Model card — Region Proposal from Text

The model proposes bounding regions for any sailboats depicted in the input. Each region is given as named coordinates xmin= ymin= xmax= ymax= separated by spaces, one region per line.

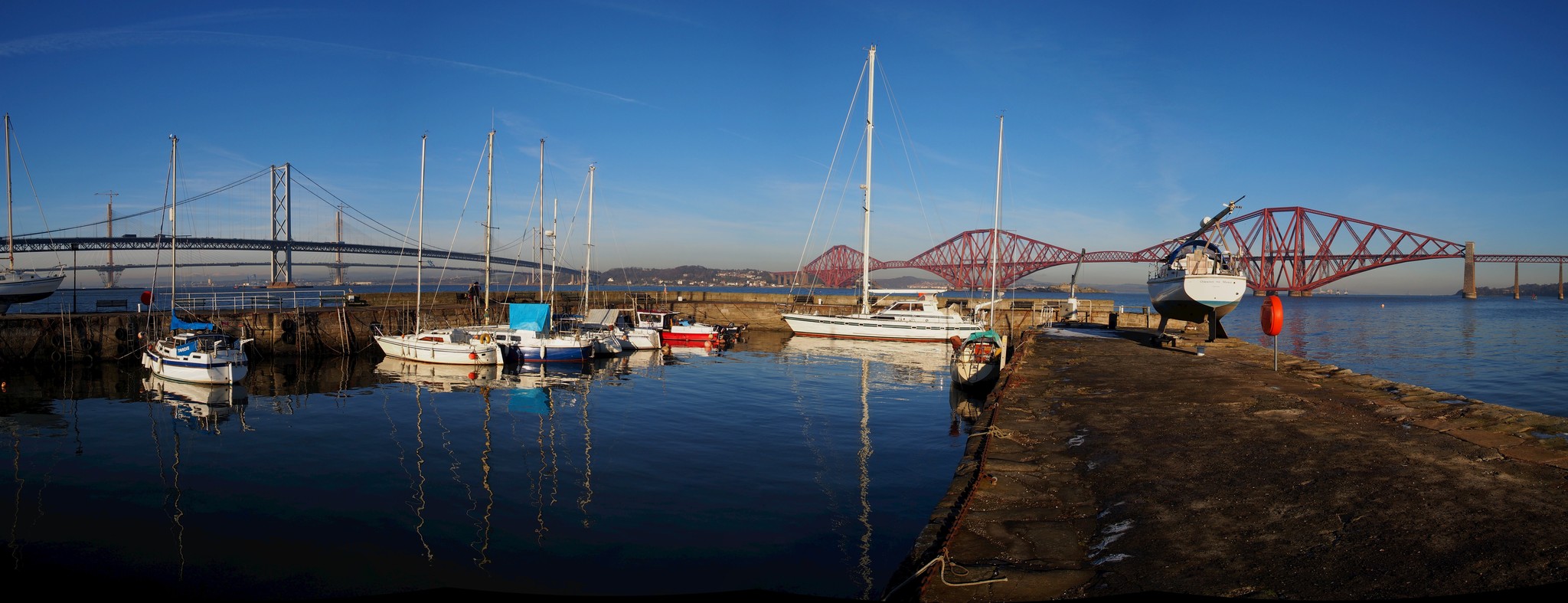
xmin=141 ymin=134 xmax=256 ymax=384
xmin=0 ymin=113 xmax=67 ymax=306
xmin=780 ymin=41 xmax=990 ymax=344
xmin=372 ymin=128 xmax=741 ymax=367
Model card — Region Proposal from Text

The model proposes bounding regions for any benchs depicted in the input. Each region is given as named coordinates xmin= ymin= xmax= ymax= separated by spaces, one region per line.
xmin=945 ymin=299 xmax=968 ymax=309
xmin=793 ymin=295 xmax=814 ymax=304
xmin=456 ymin=293 xmax=481 ymax=304
xmin=1010 ymin=301 xmax=1034 ymax=309
xmin=319 ymin=295 xmax=348 ymax=308
xmin=250 ymin=297 xmax=283 ymax=310
xmin=96 ymin=299 xmax=128 ymax=312
xmin=561 ymin=293 xmax=578 ymax=302
xmin=171 ymin=298 xmax=206 ymax=310
xmin=857 ymin=296 xmax=878 ymax=305
xmin=511 ymin=292 xmax=535 ymax=303
xmin=630 ymin=292 xmax=647 ymax=299
xmin=1044 ymin=301 xmax=1067 ymax=308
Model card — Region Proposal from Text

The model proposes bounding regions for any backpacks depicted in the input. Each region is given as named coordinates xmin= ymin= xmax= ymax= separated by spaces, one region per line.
xmin=464 ymin=293 xmax=468 ymax=299
xmin=460 ymin=294 xmax=464 ymax=299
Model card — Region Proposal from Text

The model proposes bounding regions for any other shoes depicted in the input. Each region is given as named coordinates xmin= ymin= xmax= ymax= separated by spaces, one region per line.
xmin=479 ymin=303 xmax=481 ymax=305
xmin=474 ymin=304 xmax=477 ymax=306
xmin=469 ymin=304 xmax=473 ymax=305
xmin=477 ymin=304 xmax=479 ymax=306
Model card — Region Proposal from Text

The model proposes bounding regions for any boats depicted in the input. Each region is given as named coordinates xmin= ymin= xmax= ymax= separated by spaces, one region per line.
xmin=1147 ymin=227 xmax=1248 ymax=322
xmin=949 ymin=330 xmax=1007 ymax=387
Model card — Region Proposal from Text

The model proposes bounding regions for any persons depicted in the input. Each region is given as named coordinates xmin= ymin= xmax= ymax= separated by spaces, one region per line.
xmin=468 ymin=282 xmax=481 ymax=306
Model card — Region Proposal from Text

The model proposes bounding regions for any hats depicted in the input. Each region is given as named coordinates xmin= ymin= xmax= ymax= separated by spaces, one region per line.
xmin=476 ymin=281 xmax=478 ymax=284
xmin=470 ymin=284 xmax=473 ymax=287
xmin=474 ymin=283 xmax=476 ymax=285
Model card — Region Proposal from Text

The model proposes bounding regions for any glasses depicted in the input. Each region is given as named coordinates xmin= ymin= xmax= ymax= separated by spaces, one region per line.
xmin=469 ymin=286 xmax=471 ymax=286
